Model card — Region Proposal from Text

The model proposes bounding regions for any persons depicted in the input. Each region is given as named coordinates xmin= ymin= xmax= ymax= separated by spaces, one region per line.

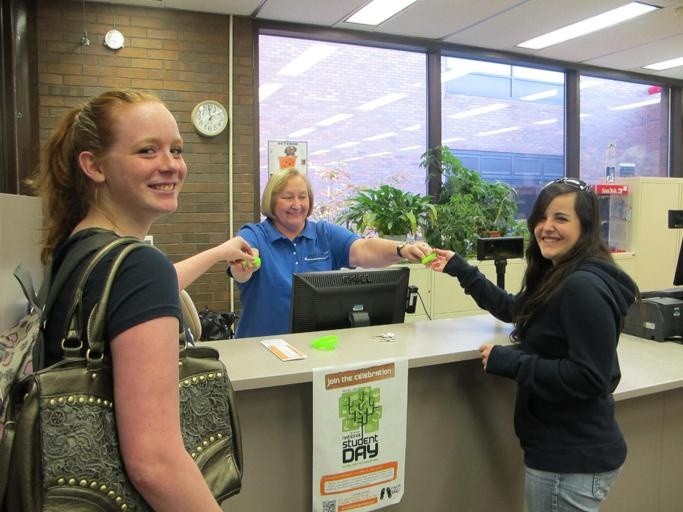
xmin=283 ymin=146 xmax=305 ymax=171
xmin=225 ymin=166 xmax=435 ymax=340
xmin=430 ymin=177 xmax=643 ymax=510
xmin=0 ymin=88 xmax=256 ymax=512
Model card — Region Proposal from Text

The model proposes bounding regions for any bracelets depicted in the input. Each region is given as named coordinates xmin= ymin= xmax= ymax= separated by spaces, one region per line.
xmin=242 ymin=254 xmax=262 ymax=270
xmin=421 ymin=251 xmax=437 ymax=265
xmin=311 ymin=335 xmax=339 ymax=351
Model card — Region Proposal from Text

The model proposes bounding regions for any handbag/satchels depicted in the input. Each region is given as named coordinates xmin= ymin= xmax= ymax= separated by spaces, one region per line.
xmin=1 ymin=234 xmax=244 ymax=512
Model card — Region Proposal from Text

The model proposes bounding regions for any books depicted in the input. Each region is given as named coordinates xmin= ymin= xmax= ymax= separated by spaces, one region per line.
xmin=260 ymin=338 xmax=307 ymax=361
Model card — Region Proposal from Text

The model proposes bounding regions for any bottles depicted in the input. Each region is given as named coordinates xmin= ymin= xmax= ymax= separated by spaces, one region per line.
xmin=605 ymin=144 xmax=615 ymax=185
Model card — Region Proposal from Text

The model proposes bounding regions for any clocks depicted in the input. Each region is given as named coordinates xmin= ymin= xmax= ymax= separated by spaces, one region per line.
xmin=192 ymin=100 xmax=228 ymax=140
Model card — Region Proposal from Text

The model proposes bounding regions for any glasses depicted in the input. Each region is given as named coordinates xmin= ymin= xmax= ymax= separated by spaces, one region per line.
xmin=538 ymin=177 xmax=593 ymax=196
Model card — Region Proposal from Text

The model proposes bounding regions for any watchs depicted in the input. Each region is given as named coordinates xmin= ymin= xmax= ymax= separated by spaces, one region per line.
xmin=397 ymin=241 xmax=409 ymax=258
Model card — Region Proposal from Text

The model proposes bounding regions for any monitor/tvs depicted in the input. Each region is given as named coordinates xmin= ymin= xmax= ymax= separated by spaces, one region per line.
xmin=291 ymin=267 xmax=409 ymax=332
